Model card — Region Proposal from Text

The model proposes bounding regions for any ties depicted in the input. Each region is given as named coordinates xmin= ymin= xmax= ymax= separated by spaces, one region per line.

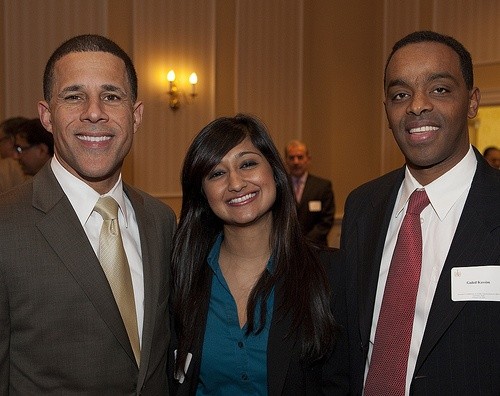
xmin=363 ymin=191 xmax=430 ymax=396
xmin=294 ymin=179 xmax=303 ymax=202
xmin=94 ymin=196 xmax=141 ymax=369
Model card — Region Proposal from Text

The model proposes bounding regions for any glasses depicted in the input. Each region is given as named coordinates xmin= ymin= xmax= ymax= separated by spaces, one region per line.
xmin=14 ymin=144 xmax=35 ymax=156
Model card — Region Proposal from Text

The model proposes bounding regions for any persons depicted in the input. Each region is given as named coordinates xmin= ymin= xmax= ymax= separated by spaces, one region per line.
xmin=0 ymin=116 xmax=34 ymax=193
xmin=162 ymin=111 xmax=343 ymax=396
xmin=11 ymin=118 xmax=55 ymax=176
xmin=282 ymin=140 xmax=335 ymax=247
xmin=330 ymin=27 xmax=500 ymax=396
xmin=0 ymin=33 xmax=181 ymax=396
xmin=482 ymin=146 xmax=500 ymax=170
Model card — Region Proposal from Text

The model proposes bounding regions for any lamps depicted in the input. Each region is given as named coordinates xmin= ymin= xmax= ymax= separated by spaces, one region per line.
xmin=165 ymin=69 xmax=199 ymax=106
